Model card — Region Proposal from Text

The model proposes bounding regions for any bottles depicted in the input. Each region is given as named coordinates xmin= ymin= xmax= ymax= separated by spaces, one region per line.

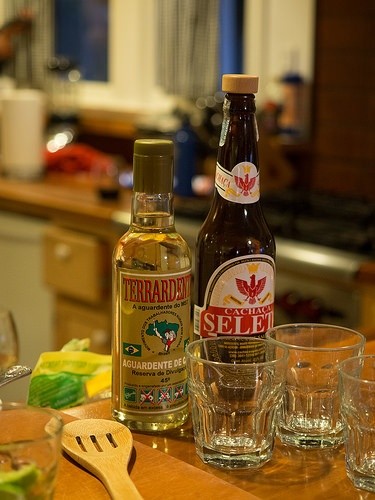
xmin=110 ymin=138 xmax=192 ymax=435
xmin=195 ymin=73 xmax=277 ymax=418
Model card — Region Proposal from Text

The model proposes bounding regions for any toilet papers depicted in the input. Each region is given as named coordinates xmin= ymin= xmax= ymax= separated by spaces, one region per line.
xmin=0 ymin=89 xmax=46 ymax=176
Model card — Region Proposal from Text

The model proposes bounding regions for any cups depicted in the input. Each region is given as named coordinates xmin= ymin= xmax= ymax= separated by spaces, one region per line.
xmin=0 ymin=310 xmax=18 ymax=373
xmin=0 ymin=403 xmax=65 ymax=500
xmin=265 ymin=323 xmax=366 ymax=450
xmin=336 ymin=354 xmax=375 ymax=494
xmin=185 ymin=337 xmax=289 ymax=470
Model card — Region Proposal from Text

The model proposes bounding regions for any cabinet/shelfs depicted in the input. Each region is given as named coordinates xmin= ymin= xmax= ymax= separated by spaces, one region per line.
xmin=39 ymin=222 xmax=114 ymax=352
